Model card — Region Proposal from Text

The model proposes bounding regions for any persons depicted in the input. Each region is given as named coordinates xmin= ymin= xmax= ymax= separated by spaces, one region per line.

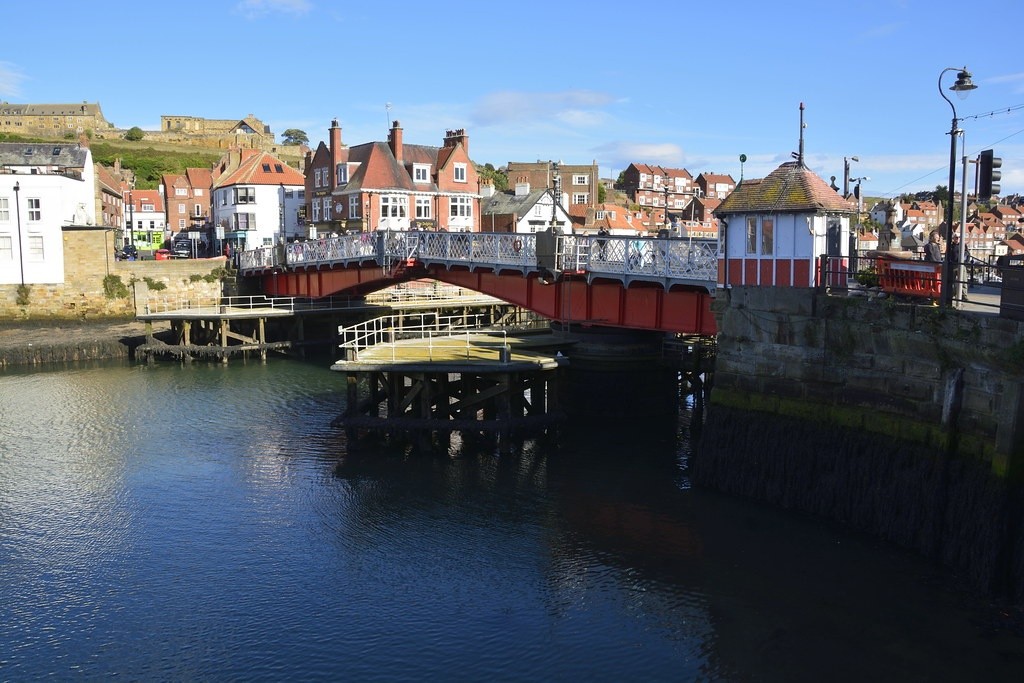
xmin=254 ymin=226 xmax=472 ymax=267
xmin=945 ymin=233 xmax=968 ymax=301
xmin=924 ymin=231 xmax=942 ymax=262
xmin=596 ymin=226 xmax=610 ymax=262
xmin=632 ymin=231 xmax=650 ymax=270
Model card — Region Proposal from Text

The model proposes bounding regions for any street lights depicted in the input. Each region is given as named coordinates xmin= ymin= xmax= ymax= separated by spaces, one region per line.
xmin=844 ymin=156 xmax=859 ymax=198
xmin=476 ymin=177 xmax=481 ymax=232
xmin=366 ymin=191 xmax=373 ymax=233
xmin=938 ymin=65 xmax=978 ymax=301
xmin=434 ymin=195 xmax=439 ymax=232
xmin=128 ymin=182 xmax=135 ymax=245
xmin=13 ymin=181 xmax=24 ymax=286
xmin=552 ymin=171 xmax=561 ymax=227
xmin=848 ymin=176 xmax=870 ymax=279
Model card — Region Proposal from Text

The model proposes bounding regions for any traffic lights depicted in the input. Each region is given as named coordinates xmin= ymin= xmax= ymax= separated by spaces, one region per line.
xmin=979 ymin=149 xmax=1002 ymax=200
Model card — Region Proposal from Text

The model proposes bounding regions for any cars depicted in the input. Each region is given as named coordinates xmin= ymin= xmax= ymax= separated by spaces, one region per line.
xmin=120 ymin=245 xmax=138 ymax=259
xmin=154 ymin=249 xmax=171 ymax=260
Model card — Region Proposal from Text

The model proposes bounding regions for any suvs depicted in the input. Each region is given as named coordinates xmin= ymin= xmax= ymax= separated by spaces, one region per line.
xmin=173 ymin=240 xmax=206 ymax=259
